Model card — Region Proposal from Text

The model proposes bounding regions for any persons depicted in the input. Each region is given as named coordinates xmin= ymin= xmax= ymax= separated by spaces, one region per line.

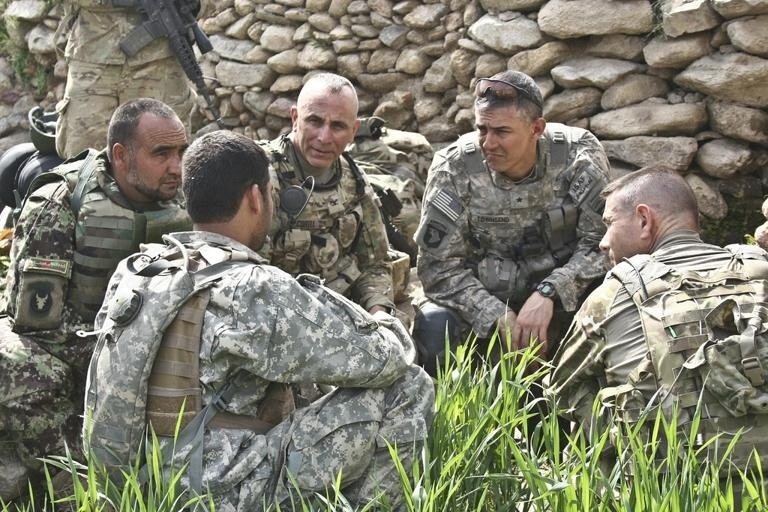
xmin=541 ymin=164 xmax=768 ymax=502
xmin=43 ymin=0 xmax=195 ymax=159
xmin=412 ymin=70 xmax=612 ymax=382
xmin=250 ymin=73 xmax=411 ymax=331
xmin=0 ymin=314 xmax=87 ymax=509
xmin=6 ymin=98 xmax=194 ymax=381
xmin=80 ymin=130 xmax=436 ymax=512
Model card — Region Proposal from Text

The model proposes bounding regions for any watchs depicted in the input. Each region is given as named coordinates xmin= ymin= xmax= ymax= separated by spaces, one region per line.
xmin=533 ymin=283 xmax=560 ymax=303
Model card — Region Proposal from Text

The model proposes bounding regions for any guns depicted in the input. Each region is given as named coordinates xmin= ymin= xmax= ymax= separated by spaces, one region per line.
xmin=114 ymin=0 xmax=224 ymax=129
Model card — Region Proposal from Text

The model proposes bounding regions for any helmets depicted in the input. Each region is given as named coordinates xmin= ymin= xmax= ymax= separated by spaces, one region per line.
xmin=26 ymin=105 xmax=58 ymax=155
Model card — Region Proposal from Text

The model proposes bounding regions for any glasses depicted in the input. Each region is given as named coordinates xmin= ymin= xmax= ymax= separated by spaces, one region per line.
xmin=473 ymin=77 xmax=542 ymax=110
